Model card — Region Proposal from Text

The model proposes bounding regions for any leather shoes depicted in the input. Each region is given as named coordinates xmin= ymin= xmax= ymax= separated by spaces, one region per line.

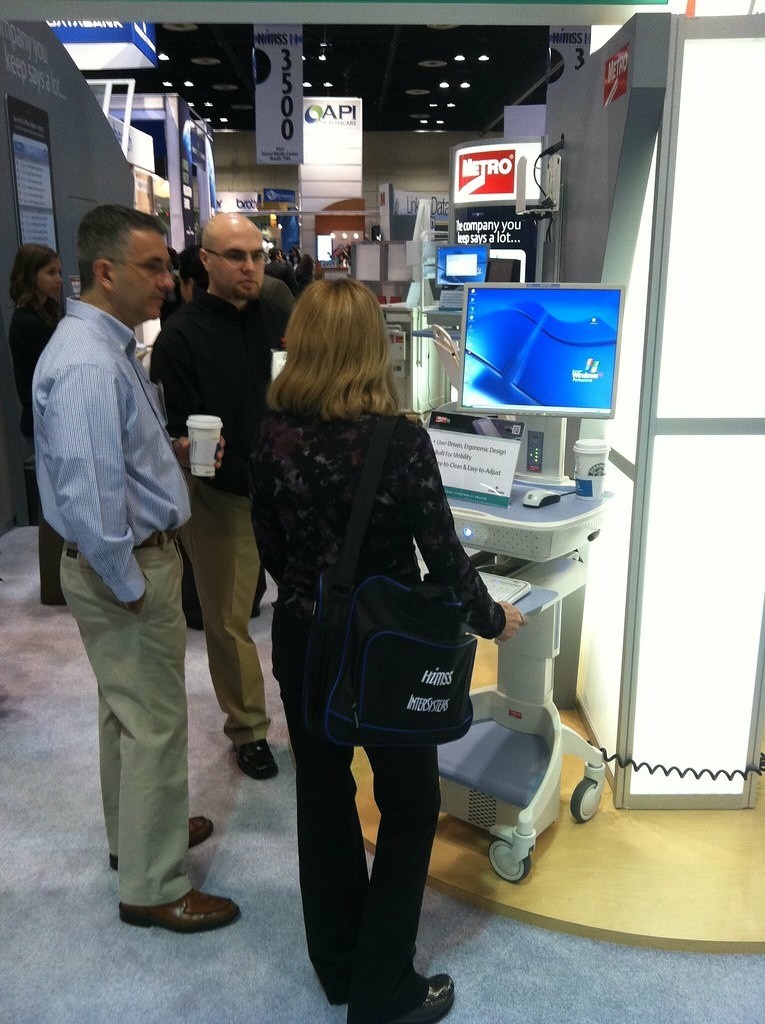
xmin=119 ymin=889 xmax=240 ymax=934
xmin=233 ymin=739 xmax=279 ymax=779
xmin=390 ymin=973 xmax=455 ymax=1024
xmin=109 ymin=815 xmax=213 ymax=870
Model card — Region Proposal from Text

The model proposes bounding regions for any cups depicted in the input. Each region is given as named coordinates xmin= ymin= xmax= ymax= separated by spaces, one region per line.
xmin=186 ymin=414 xmax=223 ymax=477
xmin=272 ymin=351 xmax=287 ymax=382
xmin=573 ymin=438 xmax=611 ymax=502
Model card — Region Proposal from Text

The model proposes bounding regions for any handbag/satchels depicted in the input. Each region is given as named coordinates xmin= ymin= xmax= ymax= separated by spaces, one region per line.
xmin=304 ymin=572 xmax=478 ymax=748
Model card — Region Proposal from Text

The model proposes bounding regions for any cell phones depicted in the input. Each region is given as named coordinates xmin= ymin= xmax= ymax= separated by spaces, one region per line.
xmin=3 ymin=94 xmax=61 ymax=256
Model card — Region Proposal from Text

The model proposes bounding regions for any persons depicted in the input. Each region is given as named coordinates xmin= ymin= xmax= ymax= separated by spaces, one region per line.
xmin=331 ymin=245 xmax=351 ymax=267
xmin=134 ymin=229 xmax=316 ymax=631
xmin=32 ymin=203 xmax=242 ymax=933
xmin=149 ymin=211 xmax=281 ymax=782
xmin=186 ymin=225 xmax=195 ymax=235
xmin=245 ymin=277 xmax=531 ymax=1024
xmin=9 ymin=241 xmax=64 ymax=609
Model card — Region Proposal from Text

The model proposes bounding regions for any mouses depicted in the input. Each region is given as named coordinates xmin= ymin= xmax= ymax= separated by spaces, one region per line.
xmin=522 ymin=489 xmax=561 ymax=508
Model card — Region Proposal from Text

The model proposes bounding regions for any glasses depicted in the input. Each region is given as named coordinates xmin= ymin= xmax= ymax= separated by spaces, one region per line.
xmin=203 ymin=248 xmax=271 ymax=267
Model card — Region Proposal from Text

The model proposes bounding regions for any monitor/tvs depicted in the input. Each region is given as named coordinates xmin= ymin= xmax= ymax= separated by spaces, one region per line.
xmin=456 ymin=282 xmax=627 ymax=419
xmin=434 ymin=243 xmax=490 ymax=288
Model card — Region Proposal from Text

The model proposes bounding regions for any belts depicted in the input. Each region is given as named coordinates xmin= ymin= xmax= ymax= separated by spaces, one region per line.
xmin=134 ymin=528 xmax=178 ymax=550
